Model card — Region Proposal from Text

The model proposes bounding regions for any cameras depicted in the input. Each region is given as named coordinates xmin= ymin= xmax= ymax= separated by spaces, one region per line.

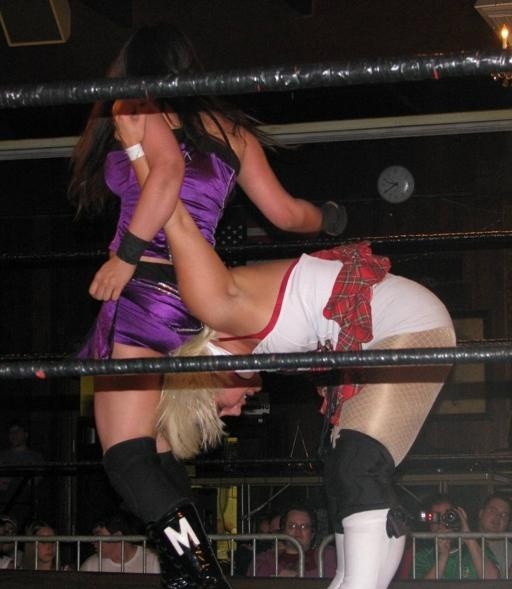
xmin=415 ymin=508 xmax=461 ymax=527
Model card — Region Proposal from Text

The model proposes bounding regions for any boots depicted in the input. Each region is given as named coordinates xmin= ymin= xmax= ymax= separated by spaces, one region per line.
xmin=145 ymin=497 xmax=232 ymax=587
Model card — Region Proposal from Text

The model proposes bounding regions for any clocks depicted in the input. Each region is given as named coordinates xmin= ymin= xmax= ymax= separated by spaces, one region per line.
xmin=377 ymin=165 xmax=415 ymax=204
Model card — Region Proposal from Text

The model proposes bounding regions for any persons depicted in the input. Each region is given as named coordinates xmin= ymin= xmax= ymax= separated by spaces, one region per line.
xmin=236 ymin=503 xmax=337 ymax=579
xmin=65 ymin=19 xmax=350 ymax=589
xmin=395 ymin=490 xmax=512 ymax=579
xmin=111 ymin=97 xmax=458 ymax=589
xmin=0 ymin=516 xmax=162 ymax=573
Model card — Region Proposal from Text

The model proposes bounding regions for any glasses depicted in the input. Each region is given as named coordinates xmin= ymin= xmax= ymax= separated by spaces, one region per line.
xmin=284 ymin=522 xmax=314 ymax=532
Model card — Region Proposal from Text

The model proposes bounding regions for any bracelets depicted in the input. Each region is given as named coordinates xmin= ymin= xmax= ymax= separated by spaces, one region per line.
xmin=123 ymin=141 xmax=145 ymax=161
xmin=117 ymin=228 xmax=150 ymax=266
